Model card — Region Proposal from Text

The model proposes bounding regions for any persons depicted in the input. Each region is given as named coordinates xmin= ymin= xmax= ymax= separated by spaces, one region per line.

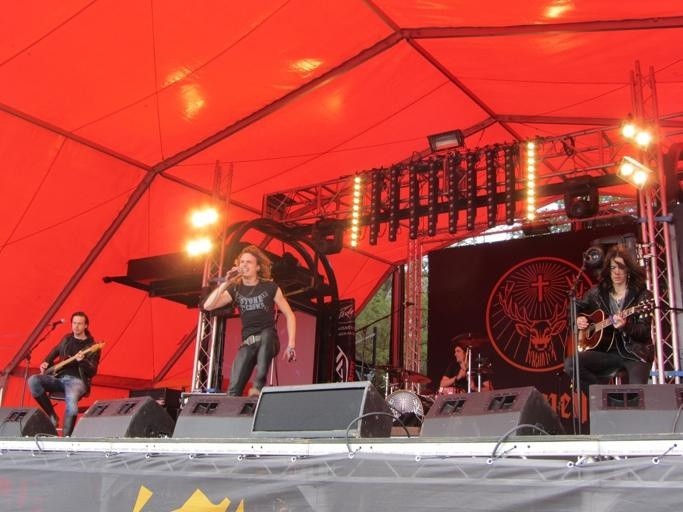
xmin=203 ymin=246 xmax=296 ymax=397
xmin=564 ymin=245 xmax=655 ymax=400
xmin=440 ymin=343 xmax=494 ymax=392
xmin=28 ymin=312 xmax=101 ymax=437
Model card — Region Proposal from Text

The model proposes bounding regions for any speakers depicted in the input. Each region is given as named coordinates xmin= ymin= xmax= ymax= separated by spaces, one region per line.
xmin=68 ymin=397 xmax=175 ymax=438
xmin=420 ymin=385 xmax=563 ymax=437
xmin=588 ymin=383 xmax=683 ymax=435
xmin=251 ymin=381 xmax=393 ymax=439
xmin=172 ymin=395 xmax=260 ymax=439
xmin=0 ymin=406 xmax=58 ymax=436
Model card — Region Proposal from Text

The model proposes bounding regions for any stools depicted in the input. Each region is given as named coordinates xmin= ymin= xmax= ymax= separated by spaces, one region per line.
xmin=50 ymin=394 xmax=67 ymax=437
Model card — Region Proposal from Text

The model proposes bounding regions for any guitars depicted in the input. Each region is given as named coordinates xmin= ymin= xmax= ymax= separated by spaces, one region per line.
xmin=565 ymin=295 xmax=654 ymax=358
xmin=43 ymin=340 xmax=105 ymax=375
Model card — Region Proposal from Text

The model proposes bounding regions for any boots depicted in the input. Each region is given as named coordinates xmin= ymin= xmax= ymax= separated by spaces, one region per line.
xmin=62 ymin=415 xmax=77 ymax=438
xmin=34 ymin=391 xmax=59 ymax=429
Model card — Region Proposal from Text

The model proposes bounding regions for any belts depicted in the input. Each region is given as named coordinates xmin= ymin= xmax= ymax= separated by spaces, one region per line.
xmin=239 ymin=335 xmax=261 ymax=348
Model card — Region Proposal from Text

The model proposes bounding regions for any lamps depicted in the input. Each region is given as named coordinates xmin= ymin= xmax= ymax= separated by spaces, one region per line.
xmin=616 ymin=121 xmax=655 ymax=189
xmin=426 ymin=129 xmax=462 ymax=152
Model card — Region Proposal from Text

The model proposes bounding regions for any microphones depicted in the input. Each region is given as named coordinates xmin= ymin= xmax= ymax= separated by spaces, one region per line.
xmin=48 ymin=319 xmax=64 ymax=326
xmin=227 ymin=269 xmax=241 ymax=280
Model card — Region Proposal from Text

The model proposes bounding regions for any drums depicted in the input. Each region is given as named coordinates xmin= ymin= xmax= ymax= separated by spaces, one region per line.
xmin=386 ymin=389 xmax=434 ymax=426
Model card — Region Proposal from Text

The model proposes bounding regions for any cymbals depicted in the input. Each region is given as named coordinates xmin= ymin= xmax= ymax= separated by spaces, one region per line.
xmin=451 ymin=334 xmax=490 ymax=345
xmin=373 ymin=365 xmax=403 ymax=372
xmin=397 ymin=369 xmax=434 ymax=384
